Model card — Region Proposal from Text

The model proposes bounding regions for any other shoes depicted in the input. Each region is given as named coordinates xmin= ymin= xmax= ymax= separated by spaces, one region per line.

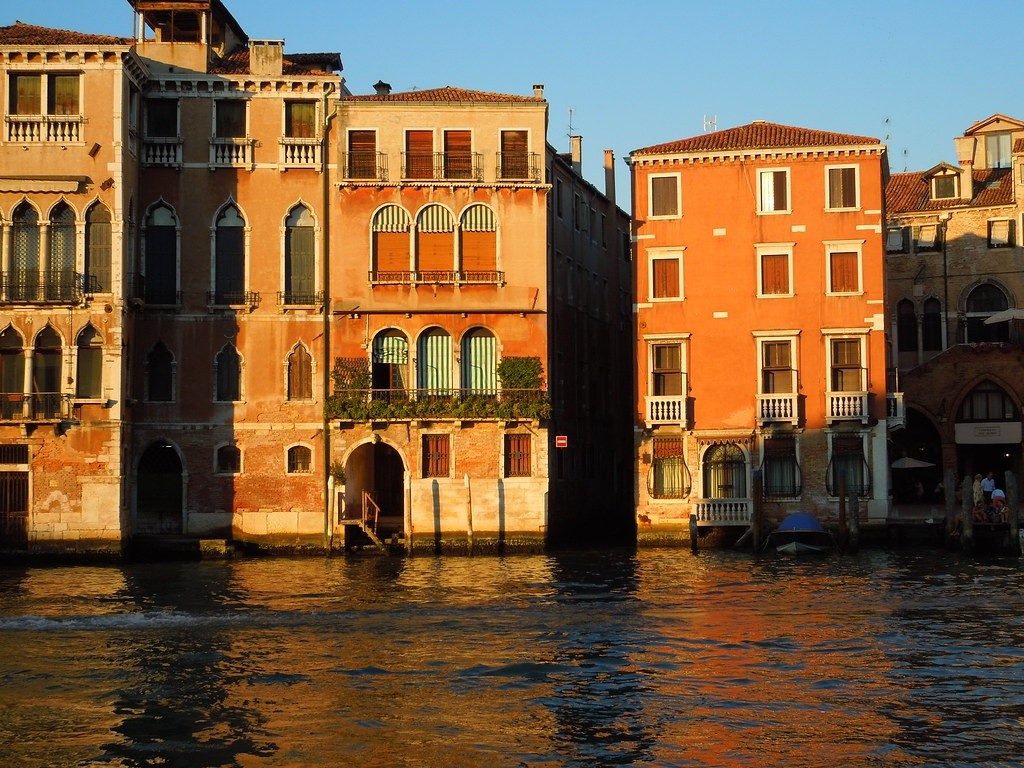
xmin=924 ymin=518 xmax=934 ymax=523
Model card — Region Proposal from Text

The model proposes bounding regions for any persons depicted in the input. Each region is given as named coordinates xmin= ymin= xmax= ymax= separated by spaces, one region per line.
xmin=949 ymin=471 xmax=1006 ymax=536
xmin=912 ymin=478 xmax=924 ymax=506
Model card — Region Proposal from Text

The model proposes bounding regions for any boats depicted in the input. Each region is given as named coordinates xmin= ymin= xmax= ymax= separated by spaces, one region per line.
xmin=771 ymin=514 xmax=834 ymax=556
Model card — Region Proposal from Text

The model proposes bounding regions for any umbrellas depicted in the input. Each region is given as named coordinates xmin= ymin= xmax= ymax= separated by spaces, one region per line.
xmin=890 ymin=456 xmax=936 ymax=468
